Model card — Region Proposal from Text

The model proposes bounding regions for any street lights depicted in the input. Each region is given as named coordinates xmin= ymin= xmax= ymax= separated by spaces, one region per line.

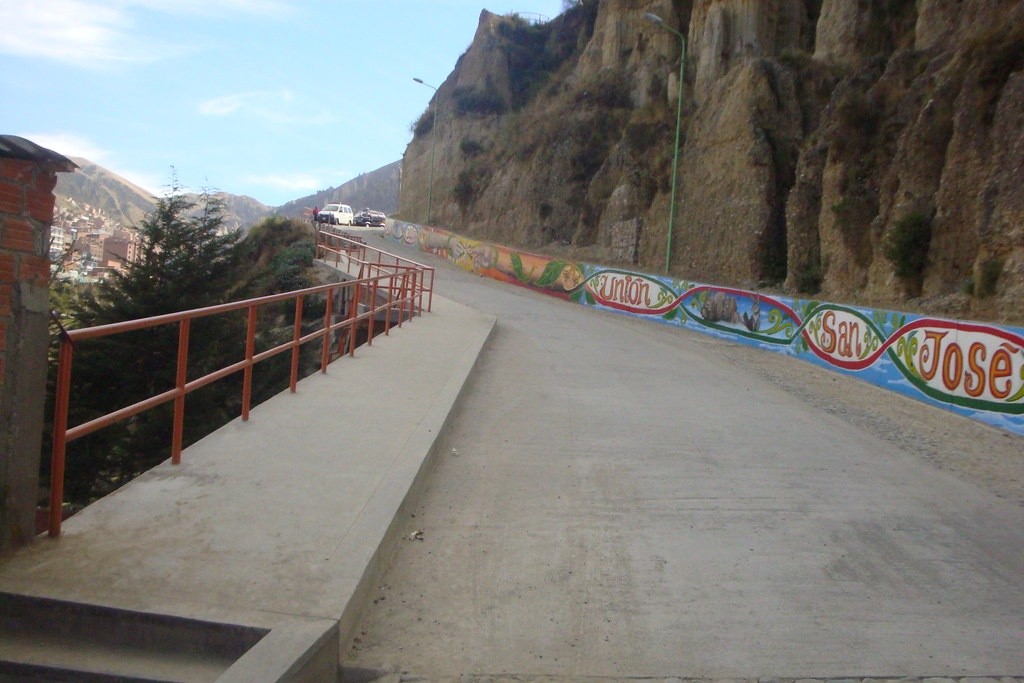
xmin=643 ymin=12 xmax=685 ymax=277
xmin=412 ymin=77 xmax=439 ymax=226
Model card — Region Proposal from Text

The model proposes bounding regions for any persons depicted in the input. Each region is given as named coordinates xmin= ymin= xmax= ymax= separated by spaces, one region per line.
xmin=313 ymin=207 xmax=318 ymax=221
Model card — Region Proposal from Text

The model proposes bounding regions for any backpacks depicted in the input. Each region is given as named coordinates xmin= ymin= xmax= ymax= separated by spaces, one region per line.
xmin=313 ymin=210 xmax=317 ymax=214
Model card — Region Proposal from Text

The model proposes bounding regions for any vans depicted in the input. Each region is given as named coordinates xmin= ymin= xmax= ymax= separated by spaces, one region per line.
xmin=353 ymin=208 xmax=386 ymax=227
xmin=316 ymin=202 xmax=354 ymax=225
